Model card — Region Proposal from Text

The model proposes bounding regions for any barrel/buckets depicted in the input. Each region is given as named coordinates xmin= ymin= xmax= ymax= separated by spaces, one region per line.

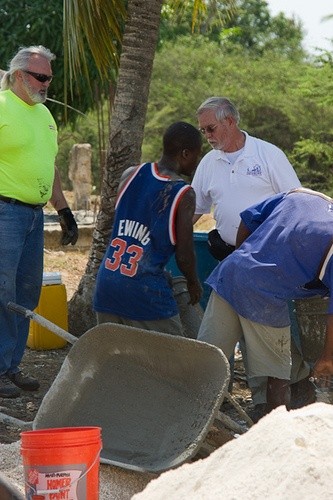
xmin=26 ymin=271 xmax=69 ymax=350
xmin=171 ymin=275 xmax=204 ymax=340
xmin=293 ymin=294 xmax=329 ymax=363
xmin=164 ymin=232 xmax=234 ymax=402
xmin=19 ymin=426 xmax=103 ymax=500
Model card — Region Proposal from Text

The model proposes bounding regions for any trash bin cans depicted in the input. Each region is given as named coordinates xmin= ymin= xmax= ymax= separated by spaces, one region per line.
xmin=164 ymin=231 xmax=219 ymax=311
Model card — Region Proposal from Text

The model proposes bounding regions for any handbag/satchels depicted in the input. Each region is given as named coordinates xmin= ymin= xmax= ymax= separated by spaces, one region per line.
xmin=206 ymin=229 xmax=236 ymax=261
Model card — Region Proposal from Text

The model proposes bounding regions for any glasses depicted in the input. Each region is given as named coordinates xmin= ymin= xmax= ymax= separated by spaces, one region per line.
xmin=199 ymin=117 xmax=228 ymax=135
xmin=21 ymin=69 xmax=53 ymax=84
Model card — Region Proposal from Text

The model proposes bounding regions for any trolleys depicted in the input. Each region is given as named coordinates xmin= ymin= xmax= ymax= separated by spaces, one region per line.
xmin=0 ymin=301 xmax=254 ymax=475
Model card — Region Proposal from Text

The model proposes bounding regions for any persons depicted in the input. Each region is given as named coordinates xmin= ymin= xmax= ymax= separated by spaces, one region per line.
xmin=198 ymin=187 xmax=333 ymax=418
xmin=0 ymin=45 xmax=79 ymax=399
xmin=190 ymin=97 xmax=333 ymax=419
xmin=94 ymin=122 xmax=203 ymax=338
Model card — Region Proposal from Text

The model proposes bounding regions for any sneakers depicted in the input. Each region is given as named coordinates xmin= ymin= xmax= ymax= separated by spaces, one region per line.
xmin=0 ymin=374 xmax=20 ymax=398
xmin=10 ymin=371 xmax=39 ymax=390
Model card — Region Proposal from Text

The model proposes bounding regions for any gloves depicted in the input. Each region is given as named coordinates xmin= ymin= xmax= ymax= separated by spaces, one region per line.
xmin=58 ymin=207 xmax=78 ymax=245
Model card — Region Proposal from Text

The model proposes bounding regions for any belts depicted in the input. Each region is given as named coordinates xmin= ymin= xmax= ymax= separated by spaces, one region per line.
xmin=0 ymin=195 xmax=44 ymax=211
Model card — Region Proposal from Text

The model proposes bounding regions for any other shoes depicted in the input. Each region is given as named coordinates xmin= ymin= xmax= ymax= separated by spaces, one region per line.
xmin=221 ymin=393 xmax=241 ymax=407
